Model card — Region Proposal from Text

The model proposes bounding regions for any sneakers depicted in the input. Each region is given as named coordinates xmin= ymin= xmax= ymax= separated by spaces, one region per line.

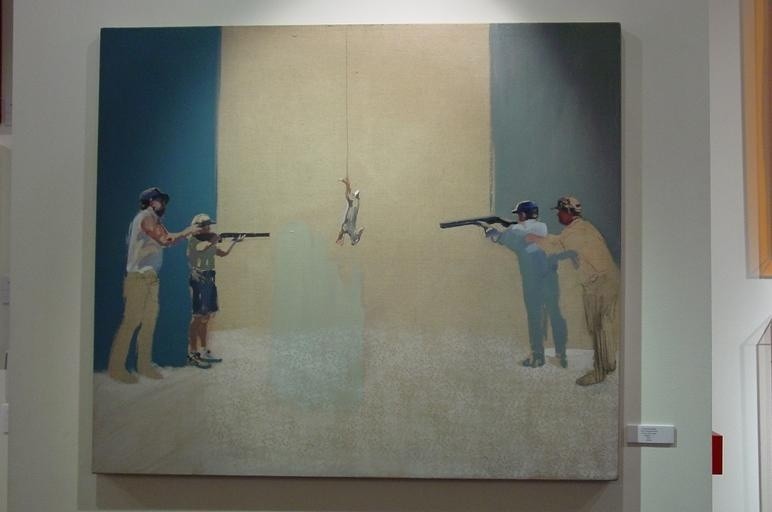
xmin=517 ymin=355 xmax=568 ymax=368
xmin=110 ymin=367 xmax=163 ymax=384
xmin=576 ymin=367 xmax=616 ymax=386
xmin=188 ymin=349 xmax=222 ymax=369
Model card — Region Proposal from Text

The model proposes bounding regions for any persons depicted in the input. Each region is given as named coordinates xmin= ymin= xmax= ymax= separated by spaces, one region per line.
xmin=185 ymin=213 xmax=249 ymax=371
xmin=106 ymin=189 xmax=204 ymax=383
xmin=524 ymin=195 xmax=619 ymax=387
xmin=470 ymin=199 xmax=571 ymax=371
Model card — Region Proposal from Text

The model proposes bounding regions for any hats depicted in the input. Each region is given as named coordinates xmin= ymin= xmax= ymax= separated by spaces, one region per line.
xmin=511 ymin=201 xmax=538 ymax=213
xmin=140 ymin=188 xmax=169 ymax=203
xmin=552 ymin=197 xmax=582 ymax=213
xmin=192 ymin=214 xmax=217 ymax=228
